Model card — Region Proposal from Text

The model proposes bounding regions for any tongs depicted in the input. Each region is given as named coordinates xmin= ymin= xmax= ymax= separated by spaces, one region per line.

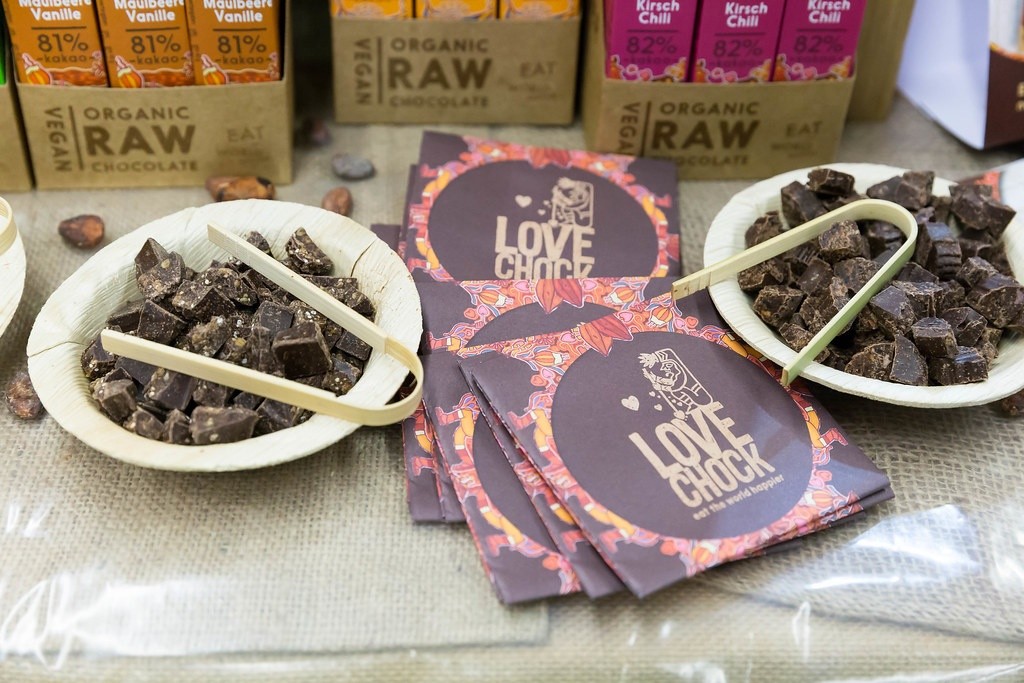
xmin=101 ymin=222 xmax=423 ymax=426
xmin=673 ymin=199 xmax=919 ymax=386
xmin=0 ymin=197 xmax=17 ymax=255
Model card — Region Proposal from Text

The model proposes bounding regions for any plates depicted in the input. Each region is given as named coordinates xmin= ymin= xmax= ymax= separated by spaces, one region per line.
xmin=703 ymin=162 xmax=1024 ymax=408
xmin=0 ymin=215 xmax=27 ymax=338
xmin=25 ymin=198 xmax=423 ymax=474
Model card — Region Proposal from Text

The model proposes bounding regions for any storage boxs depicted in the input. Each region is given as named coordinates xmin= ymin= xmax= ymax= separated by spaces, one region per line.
xmin=0 ymin=0 xmax=293 ymax=192
xmin=581 ymin=0 xmax=878 ymax=184
xmin=329 ymin=1 xmax=584 ymax=131
